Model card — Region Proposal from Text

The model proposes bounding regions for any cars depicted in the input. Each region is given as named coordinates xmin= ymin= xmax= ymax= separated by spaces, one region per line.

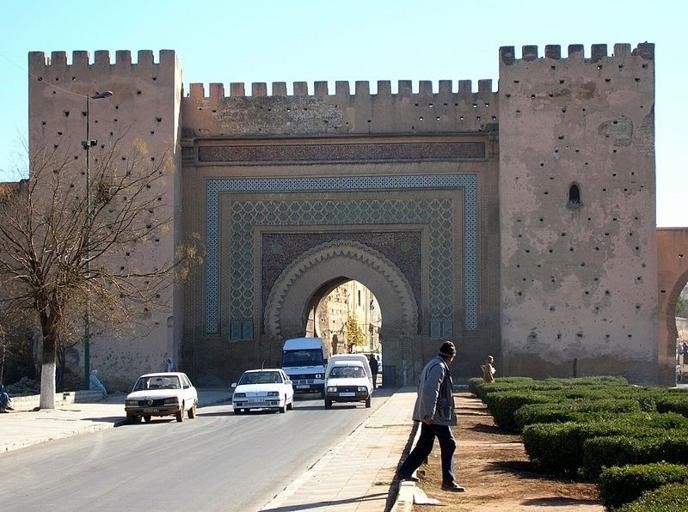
xmin=229 ymin=368 xmax=297 ymax=416
xmin=122 ymin=371 xmax=200 ymax=423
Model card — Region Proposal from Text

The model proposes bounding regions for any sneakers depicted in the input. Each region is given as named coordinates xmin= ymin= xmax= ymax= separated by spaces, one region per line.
xmin=441 ymin=482 xmax=465 ymax=493
xmin=395 ymin=473 xmax=421 ymax=484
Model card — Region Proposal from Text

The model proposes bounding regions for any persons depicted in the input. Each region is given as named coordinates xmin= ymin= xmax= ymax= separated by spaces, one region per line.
xmin=0 ymin=384 xmax=15 ymax=414
xmin=397 ymin=341 xmax=466 ymax=493
xmin=89 ymin=369 xmax=108 ymax=399
xmin=368 ymin=354 xmax=377 ymax=389
xmin=164 ymin=357 xmax=176 ymax=372
xmin=481 ymin=356 xmax=494 ymax=404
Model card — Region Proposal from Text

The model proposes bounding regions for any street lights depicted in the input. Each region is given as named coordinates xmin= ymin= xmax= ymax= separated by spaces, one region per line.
xmin=83 ymin=88 xmax=117 ymax=392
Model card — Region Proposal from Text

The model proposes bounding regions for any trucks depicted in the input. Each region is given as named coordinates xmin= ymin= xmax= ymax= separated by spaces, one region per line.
xmin=279 ymin=335 xmax=384 ymax=410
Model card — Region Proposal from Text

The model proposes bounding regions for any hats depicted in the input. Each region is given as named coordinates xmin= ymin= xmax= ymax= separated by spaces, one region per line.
xmin=438 ymin=340 xmax=457 ymax=358
